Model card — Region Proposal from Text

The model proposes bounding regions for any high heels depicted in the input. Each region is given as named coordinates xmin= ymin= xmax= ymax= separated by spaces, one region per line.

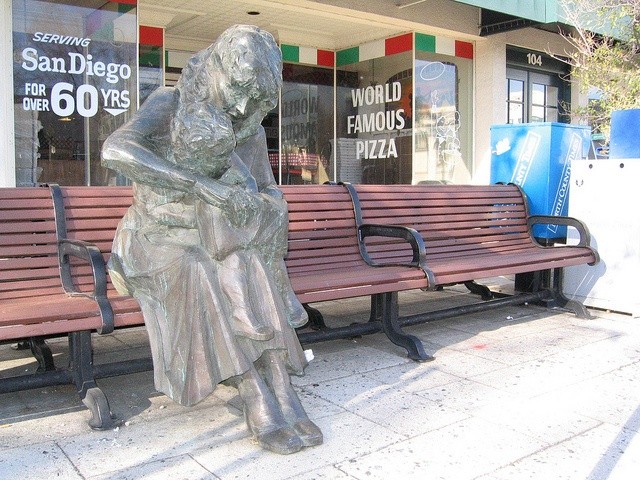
xmin=244 ymin=406 xmax=301 ymax=454
xmin=278 ymin=392 xmax=324 ymax=448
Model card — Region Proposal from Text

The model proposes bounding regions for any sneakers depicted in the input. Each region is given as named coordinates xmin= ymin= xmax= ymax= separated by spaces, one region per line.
xmin=288 ymin=303 xmax=308 ymax=328
xmin=229 ymin=316 xmax=273 ymax=340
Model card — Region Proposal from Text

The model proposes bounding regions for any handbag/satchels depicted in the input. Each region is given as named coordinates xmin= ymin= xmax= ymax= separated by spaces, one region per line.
xmin=195 ymin=198 xmax=289 ymax=260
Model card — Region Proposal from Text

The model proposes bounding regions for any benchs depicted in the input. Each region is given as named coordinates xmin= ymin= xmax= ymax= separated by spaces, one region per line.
xmin=49 ymin=186 xmax=433 ymax=433
xmin=339 ymin=180 xmax=601 ymax=362
xmin=0 ymin=185 xmax=114 ymax=431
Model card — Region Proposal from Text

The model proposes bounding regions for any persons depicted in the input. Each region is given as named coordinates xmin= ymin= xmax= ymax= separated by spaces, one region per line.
xmin=98 ymin=23 xmax=328 ymax=455
